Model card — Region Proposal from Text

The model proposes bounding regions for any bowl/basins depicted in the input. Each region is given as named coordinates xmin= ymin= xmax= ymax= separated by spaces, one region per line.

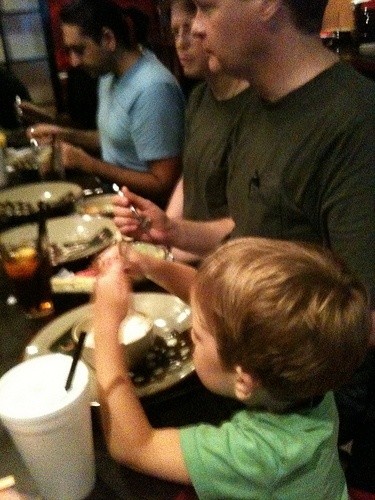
xmin=72 ymin=311 xmax=153 ymax=368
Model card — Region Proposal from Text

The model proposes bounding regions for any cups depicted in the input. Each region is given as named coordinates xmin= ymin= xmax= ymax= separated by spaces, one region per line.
xmin=0 ymin=354 xmax=96 ymax=499
xmin=32 ymin=137 xmax=64 ymax=183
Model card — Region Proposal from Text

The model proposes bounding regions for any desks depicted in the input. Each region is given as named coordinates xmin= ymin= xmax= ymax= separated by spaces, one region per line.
xmin=0 ymin=122 xmax=246 ymax=499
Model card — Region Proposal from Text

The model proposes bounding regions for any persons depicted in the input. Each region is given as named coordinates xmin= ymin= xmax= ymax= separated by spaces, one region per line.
xmin=150 ymin=1 xmax=255 ymax=260
xmin=25 ymin=1 xmax=185 ymax=207
xmin=114 ymin=1 xmax=375 ymax=305
xmin=91 ymin=236 xmax=369 ymax=499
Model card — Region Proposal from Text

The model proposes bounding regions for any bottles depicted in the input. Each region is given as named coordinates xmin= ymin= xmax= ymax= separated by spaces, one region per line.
xmin=0 ymin=134 xmax=10 ymax=184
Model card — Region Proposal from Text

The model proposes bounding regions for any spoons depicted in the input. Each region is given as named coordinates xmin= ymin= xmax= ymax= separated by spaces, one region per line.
xmin=113 ymin=184 xmax=152 ymax=233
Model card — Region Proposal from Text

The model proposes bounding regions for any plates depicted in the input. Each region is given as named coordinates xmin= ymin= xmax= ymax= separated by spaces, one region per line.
xmin=76 ymin=194 xmax=127 ymax=213
xmin=0 ymin=215 xmax=120 ymax=266
xmin=0 ymin=182 xmax=81 ymax=215
xmin=94 ymin=241 xmax=172 ymax=281
xmin=15 ymin=148 xmax=33 ymax=169
xmin=21 ymin=292 xmax=196 ymax=400
